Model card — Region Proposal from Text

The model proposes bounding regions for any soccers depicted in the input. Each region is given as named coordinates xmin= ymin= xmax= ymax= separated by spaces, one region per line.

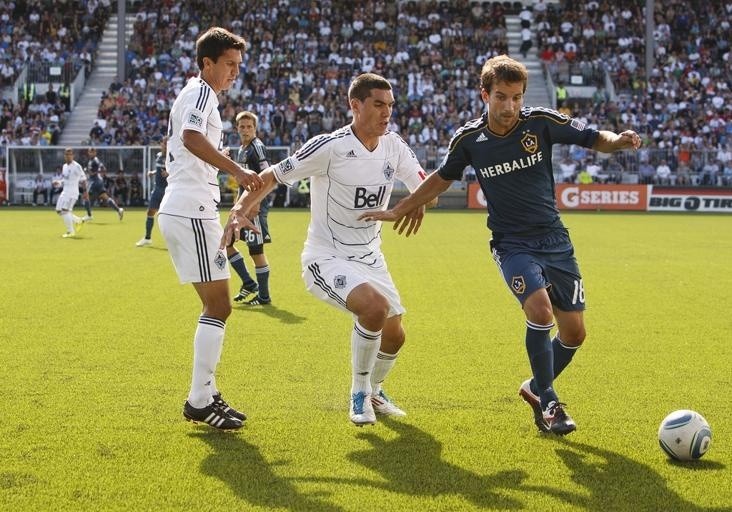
xmin=658 ymin=411 xmax=711 ymax=460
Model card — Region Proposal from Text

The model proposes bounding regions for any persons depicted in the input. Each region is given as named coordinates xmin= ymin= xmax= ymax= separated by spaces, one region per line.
xmin=520 ymin=0 xmax=732 ymax=186
xmin=83 ymin=147 xmax=123 ymax=222
xmin=1 ymin=1 xmax=110 ymax=206
xmin=356 ymin=54 xmax=645 ymax=435
xmin=53 ymin=147 xmax=90 ymax=238
xmin=227 ymin=109 xmax=272 ymax=304
xmin=157 ymin=47 xmax=262 ymax=431
xmin=218 ymin=75 xmax=441 ymax=426
xmin=79 ymin=0 xmax=520 ymax=208
xmin=137 ymin=137 xmax=170 ymax=245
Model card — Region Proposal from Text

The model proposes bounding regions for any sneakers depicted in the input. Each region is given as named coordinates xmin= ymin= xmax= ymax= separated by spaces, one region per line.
xmin=517 ymin=376 xmax=577 ymax=437
xmin=233 ymin=280 xmax=259 ymax=302
xmin=182 ymin=392 xmax=247 ymax=430
xmin=347 ymin=390 xmax=377 ymax=425
xmin=135 ymin=237 xmax=152 ymax=247
xmin=368 ymin=389 xmax=408 ymax=420
xmin=117 ymin=206 xmax=126 ymax=222
xmin=242 ymin=294 xmax=272 ymax=306
xmin=61 ymin=216 xmax=94 ymax=238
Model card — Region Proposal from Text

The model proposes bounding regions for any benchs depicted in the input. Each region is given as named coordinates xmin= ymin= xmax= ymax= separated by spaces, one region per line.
xmin=14 ymin=178 xmax=60 ymax=205
xmin=217 ymin=172 xmax=279 ymax=207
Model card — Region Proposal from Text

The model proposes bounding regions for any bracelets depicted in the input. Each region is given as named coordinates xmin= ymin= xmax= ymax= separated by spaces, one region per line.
xmin=83 ymin=190 xmax=89 ymax=192
